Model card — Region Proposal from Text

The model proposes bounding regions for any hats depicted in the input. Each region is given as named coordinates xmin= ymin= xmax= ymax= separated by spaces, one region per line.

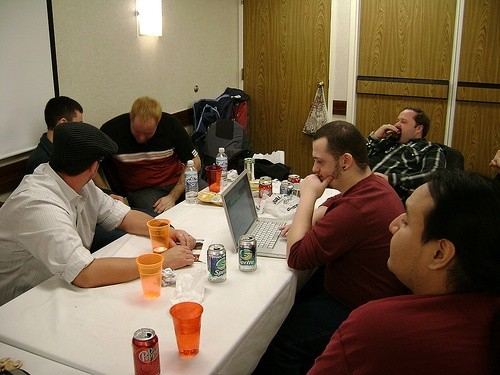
xmin=52 ymin=121 xmax=119 ymax=163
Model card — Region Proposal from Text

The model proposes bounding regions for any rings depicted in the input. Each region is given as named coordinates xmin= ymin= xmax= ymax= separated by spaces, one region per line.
xmin=178 ymin=233 xmax=184 ymax=238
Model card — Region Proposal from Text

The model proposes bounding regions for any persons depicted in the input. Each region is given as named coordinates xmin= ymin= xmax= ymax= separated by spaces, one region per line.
xmin=253 ymin=119 xmax=416 ymax=374
xmin=364 ymin=106 xmax=449 ymax=204
xmin=25 ymin=95 xmax=135 ymax=252
xmin=0 ymin=122 xmax=197 ymax=305
xmin=308 ymin=170 xmax=500 ymax=375
xmin=98 ymin=96 xmax=201 ymax=222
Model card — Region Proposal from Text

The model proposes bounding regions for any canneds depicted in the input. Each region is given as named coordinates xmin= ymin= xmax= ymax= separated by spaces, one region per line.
xmin=238 ymin=235 xmax=256 ymax=271
xmin=207 ymin=243 xmax=226 ymax=282
xmin=280 ymin=174 xmax=300 ymax=197
xmin=131 ymin=328 xmax=160 ymax=375
xmin=259 ymin=176 xmax=272 ymax=197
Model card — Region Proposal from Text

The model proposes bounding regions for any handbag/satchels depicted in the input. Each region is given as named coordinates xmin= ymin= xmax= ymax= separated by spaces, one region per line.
xmin=302 ymin=86 xmax=328 ymax=135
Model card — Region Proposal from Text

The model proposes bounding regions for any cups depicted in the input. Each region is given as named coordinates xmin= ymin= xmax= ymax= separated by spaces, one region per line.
xmin=147 ymin=218 xmax=171 ymax=254
xmin=136 ymin=253 xmax=164 ymax=300
xmin=206 ymin=165 xmax=223 ymax=193
xmin=170 ymin=301 xmax=204 ymax=358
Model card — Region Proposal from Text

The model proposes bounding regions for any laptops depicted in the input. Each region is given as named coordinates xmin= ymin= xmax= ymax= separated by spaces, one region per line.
xmin=221 ymin=169 xmax=293 ymax=259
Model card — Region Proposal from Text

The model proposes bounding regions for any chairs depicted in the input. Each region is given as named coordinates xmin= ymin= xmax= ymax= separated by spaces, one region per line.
xmin=192 ymin=99 xmax=249 ymax=175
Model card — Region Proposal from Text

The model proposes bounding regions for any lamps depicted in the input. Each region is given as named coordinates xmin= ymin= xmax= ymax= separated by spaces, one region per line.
xmin=135 ymin=0 xmax=163 ymax=37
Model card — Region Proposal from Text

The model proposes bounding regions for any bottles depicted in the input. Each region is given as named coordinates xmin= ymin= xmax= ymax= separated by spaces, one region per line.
xmin=184 ymin=160 xmax=198 ymax=204
xmin=216 ymin=148 xmax=228 ymax=187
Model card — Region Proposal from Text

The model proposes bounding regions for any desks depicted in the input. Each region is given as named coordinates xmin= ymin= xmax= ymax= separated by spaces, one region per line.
xmin=0 ymin=181 xmax=346 ymax=375
